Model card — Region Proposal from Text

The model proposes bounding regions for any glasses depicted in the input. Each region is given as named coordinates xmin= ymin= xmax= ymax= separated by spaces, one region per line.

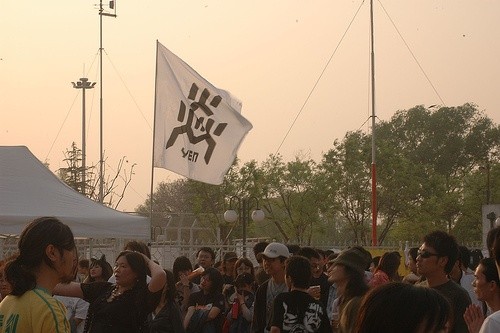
xmin=416 ymin=249 xmax=441 ymax=258
xmin=261 ymin=258 xmax=281 ymax=262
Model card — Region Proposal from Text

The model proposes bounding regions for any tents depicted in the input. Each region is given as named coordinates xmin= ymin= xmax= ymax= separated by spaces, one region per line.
xmin=0 ymin=146 xmax=151 ymax=275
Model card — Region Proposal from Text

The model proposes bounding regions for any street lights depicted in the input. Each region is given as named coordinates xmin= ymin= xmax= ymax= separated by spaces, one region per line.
xmin=224 ymin=195 xmax=265 ymax=259
xmin=71 ymin=76 xmax=96 ymax=194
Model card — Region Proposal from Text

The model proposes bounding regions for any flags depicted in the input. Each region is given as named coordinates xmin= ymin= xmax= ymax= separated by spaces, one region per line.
xmin=152 ymin=38 xmax=253 ymax=185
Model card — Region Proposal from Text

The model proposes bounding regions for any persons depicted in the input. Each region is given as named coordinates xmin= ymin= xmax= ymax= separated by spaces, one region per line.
xmin=51 ymin=248 xmax=167 ymax=333
xmin=0 ymin=220 xmax=500 ymax=333
xmin=411 ymin=228 xmax=473 ymax=333
xmin=252 ymin=242 xmax=295 ymax=333
xmin=0 ymin=217 xmax=79 ymax=333
xmin=354 ymin=282 xmax=454 ymax=333
xmin=269 ymin=254 xmax=337 ymax=333
xmin=477 ymin=225 xmax=500 ymax=333
xmin=462 ymin=255 xmax=500 ymax=333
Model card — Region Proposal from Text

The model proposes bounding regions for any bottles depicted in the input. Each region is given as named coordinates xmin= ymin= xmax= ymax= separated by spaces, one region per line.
xmin=232 ymin=300 xmax=238 ymax=319
xmin=176 ymin=265 xmax=204 ymax=286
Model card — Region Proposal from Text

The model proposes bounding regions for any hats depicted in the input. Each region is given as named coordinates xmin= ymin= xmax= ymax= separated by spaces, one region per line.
xmin=257 ymin=242 xmax=290 ymax=259
xmin=223 ymin=252 xmax=238 ymax=260
xmin=329 ymin=248 xmax=371 ymax=275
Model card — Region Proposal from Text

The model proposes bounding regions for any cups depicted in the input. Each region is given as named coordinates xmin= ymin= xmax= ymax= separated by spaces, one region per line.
xmin=310 ymin=285 xmax=321 ymax=300
